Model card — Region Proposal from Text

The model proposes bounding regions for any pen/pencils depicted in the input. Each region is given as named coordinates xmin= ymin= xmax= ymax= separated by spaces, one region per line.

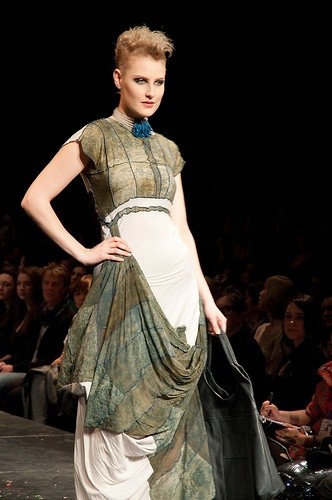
xmin=265 ymin=391 xmax=274 ymax=418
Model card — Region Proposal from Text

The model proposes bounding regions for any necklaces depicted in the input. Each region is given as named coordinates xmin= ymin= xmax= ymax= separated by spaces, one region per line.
xmin=113 ymin=107 xmax=153 ymax=137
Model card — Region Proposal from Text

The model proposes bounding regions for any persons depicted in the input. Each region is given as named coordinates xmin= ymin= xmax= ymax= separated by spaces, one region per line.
xmin=19 ymin=28 xmax=286 ymax=500
xmin=0 ymin=243 xmax=331 ymax=467
